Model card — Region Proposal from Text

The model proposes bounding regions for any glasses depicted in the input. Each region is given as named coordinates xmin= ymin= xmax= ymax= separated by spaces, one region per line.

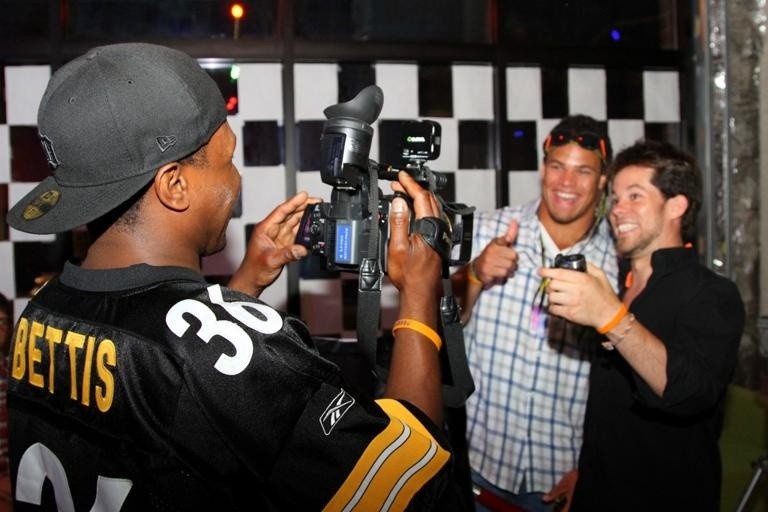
xmin=543 ymin=130 xmax=607 ymax=161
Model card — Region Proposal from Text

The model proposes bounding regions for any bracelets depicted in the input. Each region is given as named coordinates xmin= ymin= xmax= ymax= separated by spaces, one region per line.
xmin=464 ymin=256 xmax=484 ymax=287
xmin=594 ymin=301 xmax=630 ymax=335
xmin=600 ymin=312 xmax=636 ymax=351
xmin=389 ymin=318 xmax=443 ymax=354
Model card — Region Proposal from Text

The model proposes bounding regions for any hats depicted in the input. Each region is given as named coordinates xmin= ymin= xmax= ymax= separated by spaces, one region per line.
xmin=6 ymin=42 xmax=228 ymax=234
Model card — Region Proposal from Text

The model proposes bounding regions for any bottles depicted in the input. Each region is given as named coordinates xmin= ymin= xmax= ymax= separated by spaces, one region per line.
xmin=554 ymin=252 xmax=614 ymax=362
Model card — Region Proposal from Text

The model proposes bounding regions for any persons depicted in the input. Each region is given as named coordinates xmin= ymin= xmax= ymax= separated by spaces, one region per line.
xmin=1 ymin=43 xmax=454 ymax=511
xmin=535 ymin=137 xmax=746 ymax=511
xmin=446 ymin=112 xmax=622 ymax=511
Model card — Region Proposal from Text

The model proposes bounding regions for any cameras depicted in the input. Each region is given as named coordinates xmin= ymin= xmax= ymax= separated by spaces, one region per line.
xmin=295 ymin=90 xmax=473 ymax=278
